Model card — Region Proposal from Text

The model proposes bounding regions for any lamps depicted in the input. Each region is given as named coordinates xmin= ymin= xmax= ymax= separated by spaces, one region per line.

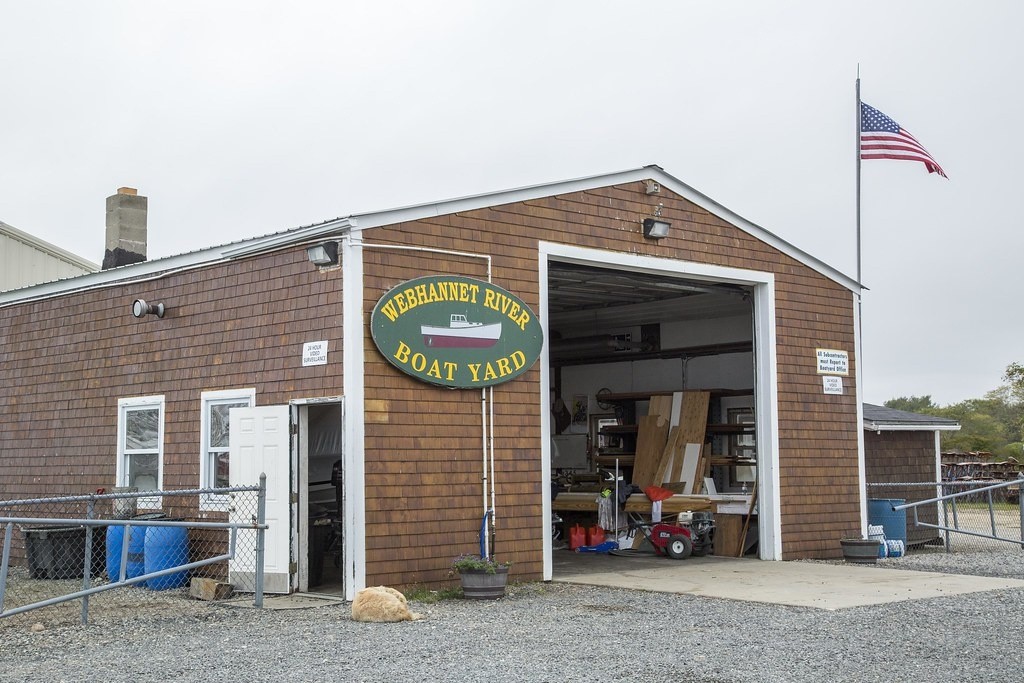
xmin=306 ymin=241 xmax=338 ymax=268
xmin=645 ymin=218 xmax=672 ymax=240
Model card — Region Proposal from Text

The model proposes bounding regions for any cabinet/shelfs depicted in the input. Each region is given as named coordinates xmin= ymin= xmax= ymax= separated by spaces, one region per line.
xmin=550 ymin=340 xmax=755 ymax=466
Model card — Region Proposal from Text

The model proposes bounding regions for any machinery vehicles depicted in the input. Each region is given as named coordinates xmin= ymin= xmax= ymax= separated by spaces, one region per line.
xmin=618 ymin=500 xmax=716 ymax=560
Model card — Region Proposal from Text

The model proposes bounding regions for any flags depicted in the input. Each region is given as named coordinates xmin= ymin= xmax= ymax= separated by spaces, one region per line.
xmin=860 ymin=99 xmax=948 ymax=180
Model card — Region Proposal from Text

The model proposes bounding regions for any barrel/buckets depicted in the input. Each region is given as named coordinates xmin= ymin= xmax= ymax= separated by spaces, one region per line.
xmin=866 ymin=498 xmax=906 ymax=558
xmin=106 ymin=512 xmax=190 ymax=591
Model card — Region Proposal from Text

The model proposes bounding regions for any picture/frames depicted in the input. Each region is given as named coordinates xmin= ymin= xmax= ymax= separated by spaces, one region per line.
xmin=571 ymin=394 xmax=590 ymax=433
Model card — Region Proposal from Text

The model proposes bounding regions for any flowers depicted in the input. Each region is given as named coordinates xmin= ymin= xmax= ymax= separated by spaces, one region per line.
xmin=448 ymin=554 xmax=513 ymax=578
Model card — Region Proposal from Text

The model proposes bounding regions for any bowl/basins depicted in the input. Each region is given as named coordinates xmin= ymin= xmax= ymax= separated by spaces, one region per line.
xmin=841 ymin=537 xmax=880 ymax=564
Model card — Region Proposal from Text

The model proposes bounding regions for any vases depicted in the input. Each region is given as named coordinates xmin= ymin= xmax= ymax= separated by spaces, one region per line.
xmin=458 ymin=567 xmax=509 ymax=601
